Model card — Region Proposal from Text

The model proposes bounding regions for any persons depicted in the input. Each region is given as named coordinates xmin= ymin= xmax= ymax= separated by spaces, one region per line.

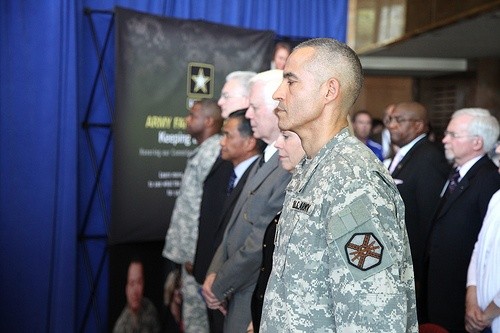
xmin=414 ymin=106 xmax=500 ymax=333
xmin=114 ymin=264 xmax=154 ymax=333
xmin=463 ymin=144 xmax=500 ymax=333
xmin=157 ymin=70 xmax=453 ymax=333
xmin=256 ymin=37 xmax=420 ymax=333
xmin=267 ymin=41 xmax=290 ymax=69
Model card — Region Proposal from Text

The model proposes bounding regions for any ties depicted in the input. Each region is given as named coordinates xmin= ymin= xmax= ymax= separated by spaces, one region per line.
xmin=256 ymin=153 xmax=265 ymax=173
xmin=227 ymin=170 xmax=237 ymax=193
xmin=447 ymin=170 xmax=460 ymax=198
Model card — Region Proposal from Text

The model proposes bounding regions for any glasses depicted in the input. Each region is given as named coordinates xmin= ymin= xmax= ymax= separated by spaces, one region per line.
xmin=389 ymin=115 xmax=419 ymax=123
xmin=443 ymin=130 xmax=474 ymax=140
